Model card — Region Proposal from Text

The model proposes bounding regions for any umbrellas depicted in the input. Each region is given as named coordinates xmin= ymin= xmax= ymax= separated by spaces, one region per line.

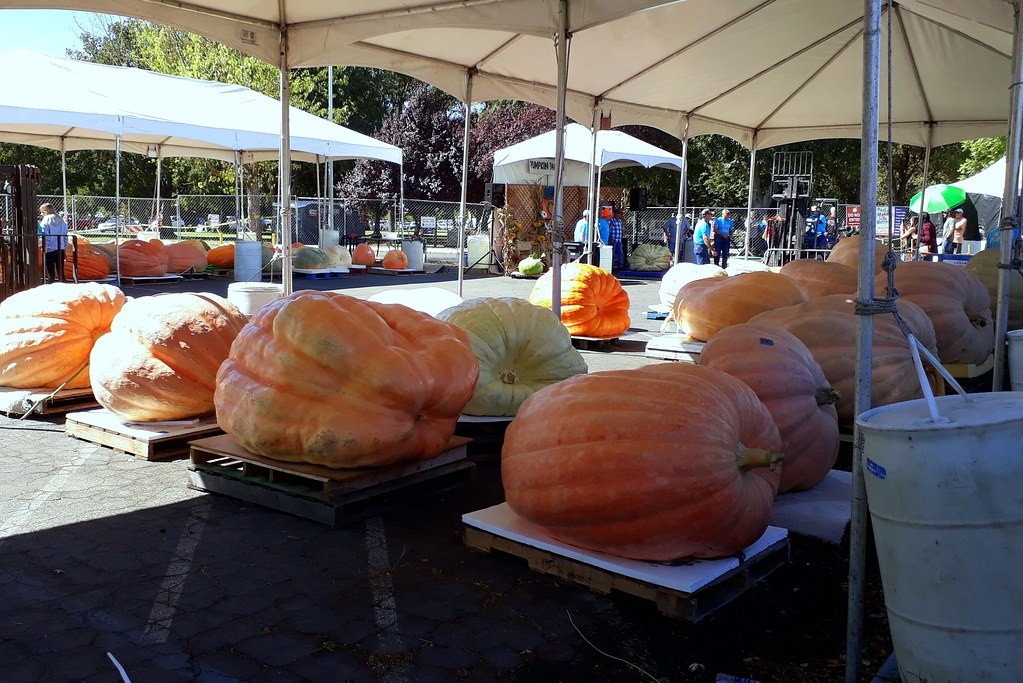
xmin=910 ymin=184 xmax=966 ymax=213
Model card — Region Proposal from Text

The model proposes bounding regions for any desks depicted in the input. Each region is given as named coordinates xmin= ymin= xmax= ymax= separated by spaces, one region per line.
xmin=564 ymin=239 xmax=613 ymax=276
xmin=924 ymin=252 xmax=974 ymax=267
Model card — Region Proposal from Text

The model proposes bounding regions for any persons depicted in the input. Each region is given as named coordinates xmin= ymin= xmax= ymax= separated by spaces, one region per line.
xmin=805 ymin=203 xmax=838 ymax=251
xmin=36 ymin=202 xmax=68 ymax=281
xmin=690 ymin=208 xmax=717 ymax=263
xmin=662 ymin=211 xmax=690 ymax=257
xmin=574 ymin=209 xmax=589 ymax=262
xmin=942 ymin=209 xmax=955 ymax=253
xmin=712 ymin=210 xmax=734 ymax=266
xmin=953 ymin=207 xmax=967 ymax=253
xmin=744 ymin=210 xmax=770 ymax=255
xmin=891 ymin=211 xmax=937 ymax=262
xmin=609 ymin=208 xmax=623 ymax=268
xmin=596 ymin=205 xmax=608 ymax=243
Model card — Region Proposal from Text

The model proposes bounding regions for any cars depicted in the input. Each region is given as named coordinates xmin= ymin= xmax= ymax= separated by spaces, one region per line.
xmin=368 ymin=216 xmax=416 ymax=230
xmin=438 ymin=218 xmax=478 ymax=230
xmin=2 ymin=209 xmax=273 ymax=244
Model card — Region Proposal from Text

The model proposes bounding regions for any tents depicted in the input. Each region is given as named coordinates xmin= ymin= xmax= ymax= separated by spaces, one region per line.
xmin=493 ymin=122 xmax=686 ymax=267
xmin=0 ymin=50 xmax=404 ymax=291
xmin=0 ymin=0 xmax=1023 ymax=682
xmin=949 ymin=153 xmax=1023 ymax=254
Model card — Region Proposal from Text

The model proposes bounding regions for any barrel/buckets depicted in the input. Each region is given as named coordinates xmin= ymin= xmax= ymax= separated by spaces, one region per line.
xmin=467 ymin=235 xmax=490 ymax=268
xmin=855 ymin=391 xmax=1023 ymax=683
xmin=1006 ymin=329 xmax=1023 ymax=391
xmin=238 ymin=232 xmax=257 ymax=241
xmin=402 ymin=237 xmax=424 ymax=271
xmin=234 ymin=241 xmax=262 ymax=282
xmin=318 ymin=229 xmax=339 ymax=250
xmin=137 ymin=231 xmax=160 ymax=242
xmin=228 ymin=282 xmax=284 ymax=323
xmin=599 ymin=244 xmax=613 ymax=275
xmin=458 ymin=252 xmax=468 ymax=267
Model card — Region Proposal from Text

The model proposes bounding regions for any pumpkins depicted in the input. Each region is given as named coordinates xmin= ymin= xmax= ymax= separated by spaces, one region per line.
xmin=0 ymin=234 xmax=408 ymax=283
xmin=0 ymin=282 xmax=588 ymax=471
xmin=498 ymin=237 xmax=1023 ymax=564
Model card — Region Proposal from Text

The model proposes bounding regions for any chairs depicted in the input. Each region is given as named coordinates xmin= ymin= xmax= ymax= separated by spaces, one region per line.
xmin=564 ymin=242 xmax=587 ymax=265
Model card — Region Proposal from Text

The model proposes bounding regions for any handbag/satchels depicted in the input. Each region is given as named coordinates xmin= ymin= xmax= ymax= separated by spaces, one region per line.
xmin=919 ymin=245 xmax=928 ymax=257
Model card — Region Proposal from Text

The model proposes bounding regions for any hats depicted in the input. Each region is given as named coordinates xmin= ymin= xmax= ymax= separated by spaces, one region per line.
xmin=955 ymin=208 xmax=963 ymax=214
xmin=601 ymin=208 xmax=613 ymax=217
xmin=701 ymin=209 xmax=714 ymax=217
xmin=722 ymin=209 xmax=730 ymax=213
xmin=942 ymin=209 xmax=951 ymax=213
xmin=583 ymin=210 xmax=590 ymax=216
xmin=614 ymin=209 xmax=622 ymax=213
